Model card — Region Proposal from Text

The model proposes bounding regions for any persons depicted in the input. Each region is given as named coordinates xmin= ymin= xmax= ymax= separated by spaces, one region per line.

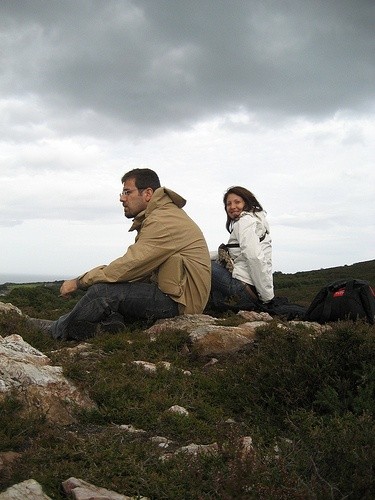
xmin=48 ymin=169 xmax=212 ymax=340
xmin=211 ymin=186 xmax=275 ymax=311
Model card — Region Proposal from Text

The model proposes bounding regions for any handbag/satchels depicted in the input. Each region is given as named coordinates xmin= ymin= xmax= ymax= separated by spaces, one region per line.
xmin=304 ymin=278 xmax=375 ymax=325
xmin=215 ymin=243 xmax=235 ymax=277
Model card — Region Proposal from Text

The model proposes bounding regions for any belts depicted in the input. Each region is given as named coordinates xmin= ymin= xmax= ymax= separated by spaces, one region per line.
xmin=241 ymin=281 xmax=258 ymax=303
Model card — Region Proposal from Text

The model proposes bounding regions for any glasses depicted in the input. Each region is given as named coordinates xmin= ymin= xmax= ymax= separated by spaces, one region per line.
xmin=119 ymin=188 xmax=141 ymax=199
